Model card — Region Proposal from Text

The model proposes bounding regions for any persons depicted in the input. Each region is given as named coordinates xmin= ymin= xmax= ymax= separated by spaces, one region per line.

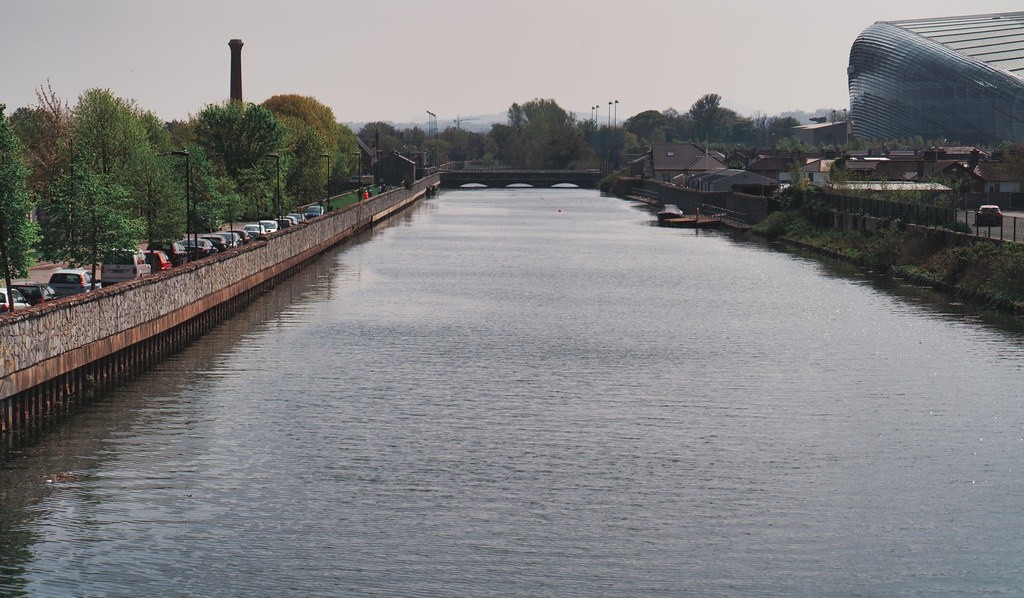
xmin=381 ymin=183 xmax=387 ymax=192
xmin=358 ymin=186 xmax=372 ymax=201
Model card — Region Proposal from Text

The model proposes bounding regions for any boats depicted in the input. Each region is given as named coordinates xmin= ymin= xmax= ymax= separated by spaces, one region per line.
xmin=657 ymin=203 xmax=683 ymax=223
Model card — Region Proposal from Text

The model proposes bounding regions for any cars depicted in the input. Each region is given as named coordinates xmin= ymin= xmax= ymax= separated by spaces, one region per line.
xmin=202 ymin=235 xmax=230 ymax=252
xmin=146 ymin=242 xmax=192 ymax=267
xmin=976 ymin=205 xmax=1003 ymax=226
xmin=48 ymin=269 xmax=103 ymax=297
xmin=228 ymin=229 xmax=252 ymax=245
xmin=291 ymin=213 xmax=308 ymax=224
xmin=257 ymin=220 xmax=281 ymax=234
xmin=279 ymin=215 xmax=298 ymax=225
xmin=143 ymin=250 xmax=174 ymax=273
xmin=182 ymin=239 xmax=218 ymax=259
xmin=307 ymin=206 xmax=323 ymax=218
xmin=218 ymin=233 xmax=244 ymax=249
xmin=11 ymin=282 xmax=61 ymax=307
xmin=243 ymin=224 xmax=270 ymax=241
xmin=275 ymin=218 xmax=292 ymax=230
xmin=0 ymin=287 xmax=33 ymax=314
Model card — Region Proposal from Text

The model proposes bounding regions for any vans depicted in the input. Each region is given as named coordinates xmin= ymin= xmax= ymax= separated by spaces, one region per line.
xmin=99 ymin=247 xmax=152 ymax=286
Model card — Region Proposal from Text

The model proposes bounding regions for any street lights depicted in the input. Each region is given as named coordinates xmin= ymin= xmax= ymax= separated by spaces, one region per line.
xmin=170 ymin=150 xmax=190 ymax=263
xmin=266 ymin=154 xmax=279 ymax=221
xmin=351 ymin=152 xmax=362 ymax=201
xmin=321 ymin=154 xmax=330 ymax=202
